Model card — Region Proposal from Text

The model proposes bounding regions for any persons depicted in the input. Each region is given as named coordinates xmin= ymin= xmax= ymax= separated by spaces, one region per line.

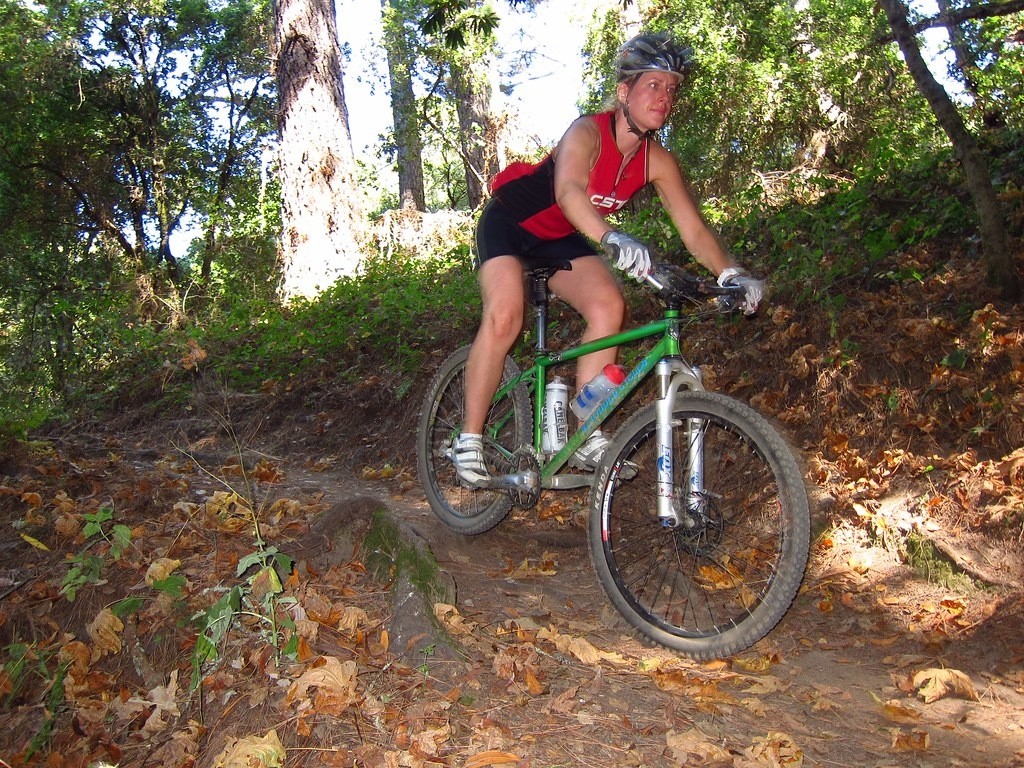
xmin=450 ymin=35 xmax=764 ymax=490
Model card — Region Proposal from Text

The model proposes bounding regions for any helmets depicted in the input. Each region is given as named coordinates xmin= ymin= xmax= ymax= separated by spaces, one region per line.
xmin=620 ymin=35 xmax=692 ymax=84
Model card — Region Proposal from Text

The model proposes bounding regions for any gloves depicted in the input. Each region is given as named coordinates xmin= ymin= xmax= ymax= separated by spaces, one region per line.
xmin=716 ymin=268 xmax=763 ymax=317
xmin=601 ymin=230 xmax=652 ymax=284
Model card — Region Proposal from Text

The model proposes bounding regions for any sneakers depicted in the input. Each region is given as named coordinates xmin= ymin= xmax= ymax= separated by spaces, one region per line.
xmin=567 ymin=435 xmax=639 ymax=480
xmin=448 ymin=436 xmax=491 ymax=489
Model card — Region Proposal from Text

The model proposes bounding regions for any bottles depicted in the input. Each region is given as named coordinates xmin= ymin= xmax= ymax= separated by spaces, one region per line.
xmin=541 ymin=375 xmax=567 ymax=454
xmin=574 ymin=363 xmax=625 ymax=421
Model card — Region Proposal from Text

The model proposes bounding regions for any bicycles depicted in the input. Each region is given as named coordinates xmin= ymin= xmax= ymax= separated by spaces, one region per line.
xmin=414 ymin=243 xmax=815 ymax=667
